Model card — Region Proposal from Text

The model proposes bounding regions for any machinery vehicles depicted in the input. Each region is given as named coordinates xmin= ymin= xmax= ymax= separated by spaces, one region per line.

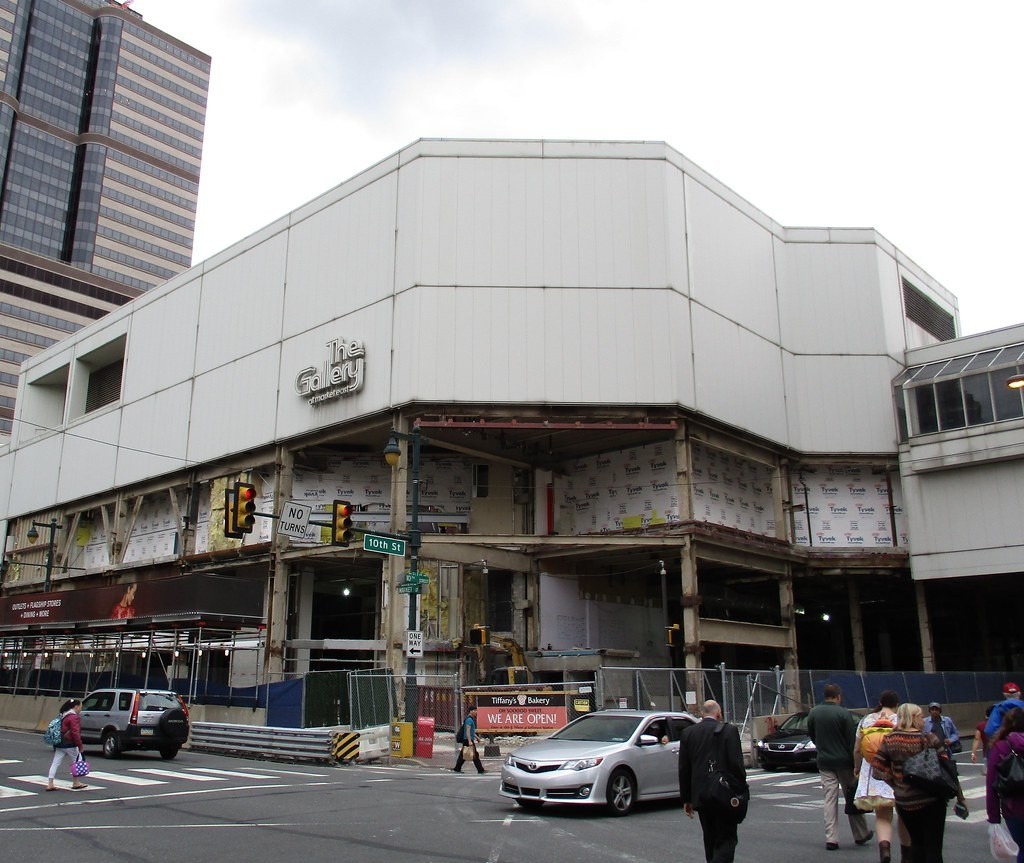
xmin=470 ymin=624 xmax=531 ymax=684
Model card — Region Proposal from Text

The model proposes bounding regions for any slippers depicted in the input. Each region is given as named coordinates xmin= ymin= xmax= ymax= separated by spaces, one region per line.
xmin=71 ymin=784 xmax=88 ymax=789
xmin=46 ymin=787 xmax=62 ymax=791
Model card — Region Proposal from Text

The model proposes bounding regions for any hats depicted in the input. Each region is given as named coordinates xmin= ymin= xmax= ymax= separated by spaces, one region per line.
xmin=1003 ymin=682 xmax=1020 ymax=694
xmin=929 ymin=702 xmax=941 ymax=709
xmin=650 ymin=722 xmax=660 ymax=730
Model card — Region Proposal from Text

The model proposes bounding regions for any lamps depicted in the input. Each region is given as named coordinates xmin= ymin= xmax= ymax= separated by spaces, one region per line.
xmin=659 ymin=560 xmax=666 ymax=575
xmin=789 ymin=603 xmax=804 ymax=614
xmin=462 ymin=559 xmax=488 ymax=574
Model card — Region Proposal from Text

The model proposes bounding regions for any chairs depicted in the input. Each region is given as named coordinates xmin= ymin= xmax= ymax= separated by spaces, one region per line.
xmin=644 ymin=724 xmax=660 ymax=746
xmin=101 ymin=698 xmax=114 ymax=711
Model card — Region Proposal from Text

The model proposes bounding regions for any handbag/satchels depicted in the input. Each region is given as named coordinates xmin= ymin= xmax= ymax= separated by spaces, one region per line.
xmin=988 ymin=817 xmax=1020 ymax=863
xmin=950 ymin=722 xmax=962 ymax=753
xmin=693 ymin=770 xmax=749 ymax=824
xmin=902 ymin=731 xmax=959 ymax=799
xmin=845 ymin=780 xmax=874 ymax=815
xmin=70 ymin=752 xmax=90 ymax=777
xmin=462 ymin=746 xmax=475 ymax=761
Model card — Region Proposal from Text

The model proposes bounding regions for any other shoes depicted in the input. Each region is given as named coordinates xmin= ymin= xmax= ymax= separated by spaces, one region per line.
xmin=826 ymin=843 xmax=839 ymax=850
xmin=478 ymin=771 xmax=489 ymax=775
xmin=855 ymin=830 xmax=873 ymax=844
xmin=453 ymin=769 xmax=464 ymax=774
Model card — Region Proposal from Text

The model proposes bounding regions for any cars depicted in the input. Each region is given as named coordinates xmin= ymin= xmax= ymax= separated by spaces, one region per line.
xmin=498 ymin=709 xmax=701 ymax=816
xmin=732 ymin=723 xmax=751 ymax=753
xmin=757 ymin=709 xmax=864 ymax=772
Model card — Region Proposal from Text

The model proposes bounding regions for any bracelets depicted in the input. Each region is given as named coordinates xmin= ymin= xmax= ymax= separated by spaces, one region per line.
xmin=972 ymin=753 xmax=976 ymax=755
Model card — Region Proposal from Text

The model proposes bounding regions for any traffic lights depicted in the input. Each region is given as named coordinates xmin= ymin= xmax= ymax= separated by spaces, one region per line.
xmin=232 ymin=482 xmax=256 ymax=533
xmin=332 ymin=499 xmax=353 ymax=547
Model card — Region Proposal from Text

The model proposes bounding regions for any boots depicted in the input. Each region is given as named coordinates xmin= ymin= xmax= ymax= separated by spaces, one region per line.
xmin=879 ymin=840 xmax=891 ymax=863
xmin=900 ymin=843 xmax=912 ymax=863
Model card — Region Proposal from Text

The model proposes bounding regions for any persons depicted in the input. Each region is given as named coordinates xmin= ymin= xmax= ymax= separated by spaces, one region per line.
xmin=971 ymin=705 xmax=994 ymax=775
xmin=47 ymin=698 xmax=88 ymax=790
xmin=853 ymin=690 xmax=912 ymax=863
xmin=923 ymin=702 xmax=959 ymax=746
xmin=644 ymin=720 xmax=668 ymax=744
xmin=986 ymin=707 xmax=1024 ymax=863
xmin=454 ymin=706 xmax=487 ymax=773
xmin=808 ymin=683 xmax=873 ymax=849
xmin=679 ymin=700 xmax=746 ymax=863
xmin=110 ymin=582 xmax=137 ymax=619
xmin=873 ymin=704 xmax=964 ymax=863
xmin=984 ymin=682 xmax=1024 ymax=737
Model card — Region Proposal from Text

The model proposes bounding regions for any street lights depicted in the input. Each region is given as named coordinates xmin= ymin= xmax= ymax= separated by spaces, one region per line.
xmin=384 ymin=425 xmax=422 ymax=755
xmin=27 ymin=518 xmax=63 ymax=593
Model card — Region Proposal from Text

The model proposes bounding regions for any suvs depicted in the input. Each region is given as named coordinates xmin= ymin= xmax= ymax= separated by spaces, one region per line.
xmin=54 ymin=689 xmax=189 ymax=760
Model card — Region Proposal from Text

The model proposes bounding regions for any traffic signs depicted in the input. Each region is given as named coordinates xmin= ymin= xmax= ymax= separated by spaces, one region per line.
xmin=363 ymin=534 xmax=405 ymax=556
xmin=407 ymin=630 xmax=424 ymax=658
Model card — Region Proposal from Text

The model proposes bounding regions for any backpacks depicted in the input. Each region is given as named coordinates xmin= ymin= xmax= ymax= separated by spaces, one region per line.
xmin=43 ymin=713 xmax=62 ymax=745
xmin=456 ymin=726 xmax=464 ymax=743
xmin=991 ymin=740 xmax=1024 ymax=798
